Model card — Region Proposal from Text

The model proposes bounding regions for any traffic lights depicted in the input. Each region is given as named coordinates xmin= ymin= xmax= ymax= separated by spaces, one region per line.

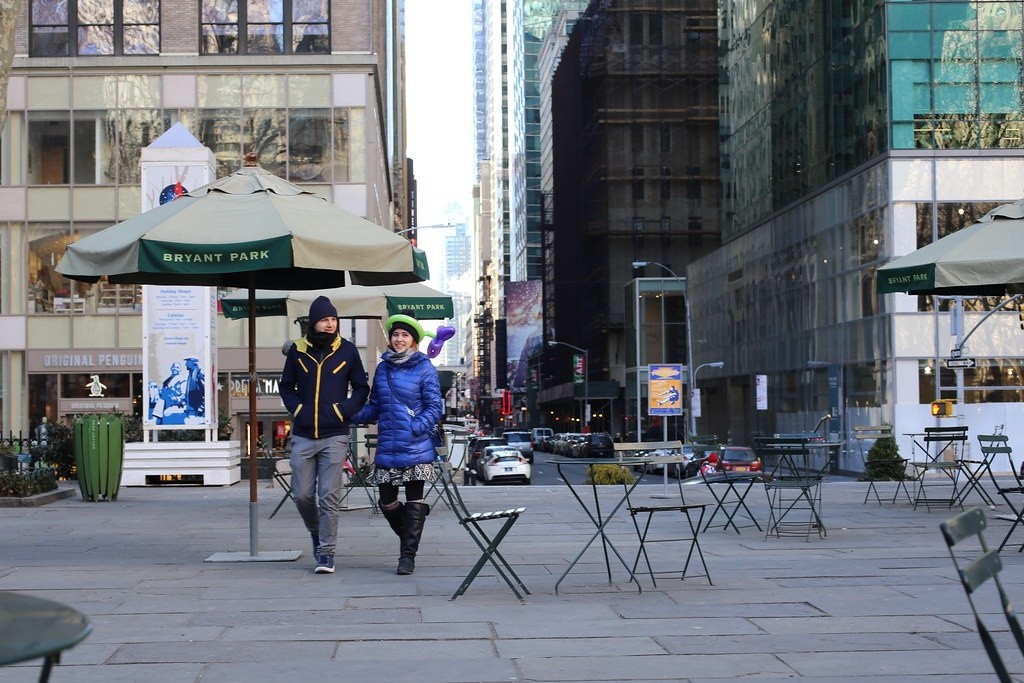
xmin=931 ymin=400 xmax=953 ymax=417
xmin=1018 ymin=303 xmax=1024 ymax=330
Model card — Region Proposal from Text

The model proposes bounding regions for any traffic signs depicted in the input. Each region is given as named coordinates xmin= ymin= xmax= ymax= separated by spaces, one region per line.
xmin=947 ymin=359 xmax=976 ymax=368
xmin=950 ymin=350 xmax=963 ymax=357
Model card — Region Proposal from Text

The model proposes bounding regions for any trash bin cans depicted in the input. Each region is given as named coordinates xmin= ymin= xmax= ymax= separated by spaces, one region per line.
xmin=71 ymin=411 xmax=127 ymax=503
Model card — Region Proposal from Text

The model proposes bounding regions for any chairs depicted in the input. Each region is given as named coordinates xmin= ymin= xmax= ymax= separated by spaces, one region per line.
xmin=431 ymin=443 xmax=533 ymax=605
xmin=954 ymin=424 xmax=1005 ymax=508
xmin=365 ymin=433 xmax=387 ymax=515
xmin=851 ymin=423 xmax=914 ymax=504
xmin=257 ymin=434 xmax=294 ymax=520
xmin=773 ymin=431 xmax=821 ymax=529
xmin=761 ymin=439 xmax=830 ymax=544
xmin=906 ymin=424 xmax=968 ymax=513
xmin=976 ymin=431 xmax=1024 ymax=555
xmin=691 ymin=432 xmax=763 ymax=534
xmin=613 ymin=436 xmax=716 ymax=587
xmin=424 ymin=428 xmax=472 ymax=512
xmin=933 ymin=506 xmax=1024 ymax=683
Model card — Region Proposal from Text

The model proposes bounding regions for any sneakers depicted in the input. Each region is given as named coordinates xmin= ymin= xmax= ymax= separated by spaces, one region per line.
xmin=313 ymin=543 xmax=319 ymax=561
xmin=315 ymin=555 xmax=335 ymax=573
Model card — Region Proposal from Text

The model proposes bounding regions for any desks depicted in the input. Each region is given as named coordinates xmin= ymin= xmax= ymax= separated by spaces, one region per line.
xmin=0 ymin=588 xmax=92 ymax=683
xmin=902 ymin=430 xmax=969 ymax=502
xmin=757 ymin=441 xmax=846 ymax=537
xmin=349 ymin=435 xmax=383 ymax=518
xmin=545 ymin=459 xmax=654 ymax=597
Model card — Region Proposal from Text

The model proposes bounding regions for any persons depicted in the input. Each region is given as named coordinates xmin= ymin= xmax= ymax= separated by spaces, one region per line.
xmin=279 ymin=295 xmax=370 ymax=574
xmin=658 ymin=386 xmax=679 ymax=405
xmin=614 ymin=432 xmax=622 ymax=443
xmin=161 ymin=357 xmax=206 ymax=418
xmin=350 ymin=309 xmax=443 ymax=576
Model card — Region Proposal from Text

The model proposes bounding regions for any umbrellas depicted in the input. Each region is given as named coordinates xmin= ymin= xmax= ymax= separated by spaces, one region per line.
xmin=876 ymin=196 xmax=1024 ymax=297
xmin=220 ymin=288 xmax=454 ymax=487
xmin=53 ymin=152 xmax=430 ymax=562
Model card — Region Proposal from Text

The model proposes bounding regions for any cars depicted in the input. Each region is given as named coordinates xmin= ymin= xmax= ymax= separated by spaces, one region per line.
xmin=463 ymin=417 xmax=762 ymax=485
xmin=482 ymin=450 xmax=534 ymax=485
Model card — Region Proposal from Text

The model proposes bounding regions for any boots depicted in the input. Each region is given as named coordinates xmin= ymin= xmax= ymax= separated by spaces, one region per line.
xmin=377 ymin=499 xmax=402 ymax=537
xmin=397 ymin=501 xmax=431 ymax=574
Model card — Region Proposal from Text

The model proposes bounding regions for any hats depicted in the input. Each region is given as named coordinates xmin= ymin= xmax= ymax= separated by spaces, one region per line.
xmin=309 ymin=295 xmax=339 ymax=326
xmin=389 ymin=309 xmax=419 ymax=343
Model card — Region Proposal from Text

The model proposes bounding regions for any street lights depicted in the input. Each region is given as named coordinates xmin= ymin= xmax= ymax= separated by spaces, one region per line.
xmin=548 ymin=341 xmax=588 ymax=426
xmin=633 ymin=261 xmax=724 ymax=443
